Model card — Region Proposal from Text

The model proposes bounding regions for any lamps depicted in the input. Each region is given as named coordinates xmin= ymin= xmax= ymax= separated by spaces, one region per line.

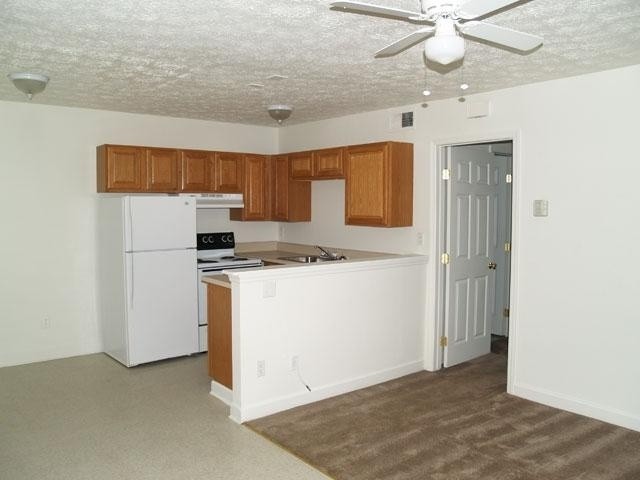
xmin=266 ymin=105 xmax=293 ymax=124
xmin=7 ymin=72 xmax=49 ymax=99
xmin=423 ymin=27 xmax=468 ymax=98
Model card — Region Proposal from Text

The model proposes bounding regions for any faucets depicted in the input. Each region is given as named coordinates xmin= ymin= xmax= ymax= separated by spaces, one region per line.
xmin=314 ymin=245 xmax=330 ymax=256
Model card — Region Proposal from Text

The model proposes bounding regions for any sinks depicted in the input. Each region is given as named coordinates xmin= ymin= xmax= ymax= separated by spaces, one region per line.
xmin=278 ymin=255 xmax=322 ymax=263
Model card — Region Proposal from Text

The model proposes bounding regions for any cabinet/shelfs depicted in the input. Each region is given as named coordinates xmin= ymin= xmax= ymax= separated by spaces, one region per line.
xmin=346 ymin=142 xmax=412 ymax=228
xmin=97 ymin=145 xmax=181 ymax=193
xmin=231 ymin=152 xmax=271 ymax=221
xmin=289 ymin=149 xmax=345 ymax=179
xmin=269 ymin=155 xmax=312 ymax=223
xmin=181 ymin=148 xmax=242 ymax=194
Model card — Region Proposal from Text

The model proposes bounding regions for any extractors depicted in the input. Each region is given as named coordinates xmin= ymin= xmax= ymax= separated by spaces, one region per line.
xmin=178 ymin=192 xmax=245 ymax=209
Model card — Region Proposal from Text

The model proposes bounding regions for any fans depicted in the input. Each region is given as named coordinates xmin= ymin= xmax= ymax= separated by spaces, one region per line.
xmin=330 ymin=0 xmax=542 ymax=55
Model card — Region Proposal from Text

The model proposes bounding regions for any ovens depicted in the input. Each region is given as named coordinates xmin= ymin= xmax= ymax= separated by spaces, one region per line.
xmin=197 ymin=267 xmax=264 ymax=326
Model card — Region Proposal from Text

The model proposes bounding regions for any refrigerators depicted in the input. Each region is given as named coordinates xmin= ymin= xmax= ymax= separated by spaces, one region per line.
xmin=101 ymin=196 xmax=198 ymax=368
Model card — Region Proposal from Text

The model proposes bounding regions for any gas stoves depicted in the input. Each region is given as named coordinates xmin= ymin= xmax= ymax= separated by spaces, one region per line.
xmin=197 ymin=255 xmax=261 ymax=270
